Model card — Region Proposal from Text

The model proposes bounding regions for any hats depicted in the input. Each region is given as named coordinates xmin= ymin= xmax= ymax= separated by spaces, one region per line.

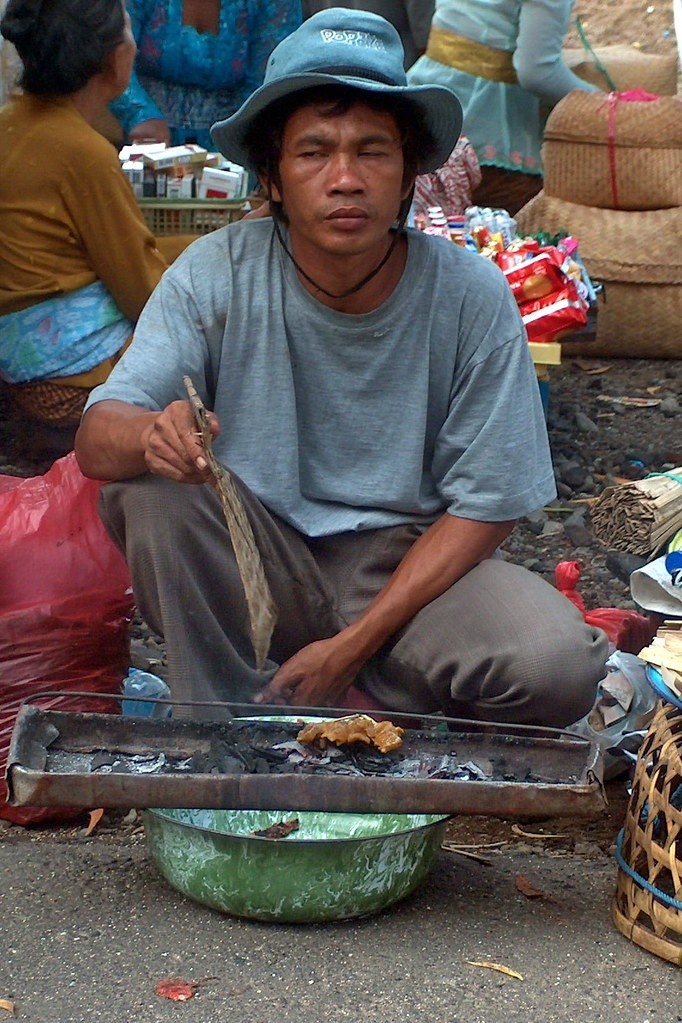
xmin=209 ymin=7 xmax=464 ymax=178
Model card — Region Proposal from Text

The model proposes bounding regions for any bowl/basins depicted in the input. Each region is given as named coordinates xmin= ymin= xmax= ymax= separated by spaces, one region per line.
xmin=139 ymin=713 xmax=459 ymax=925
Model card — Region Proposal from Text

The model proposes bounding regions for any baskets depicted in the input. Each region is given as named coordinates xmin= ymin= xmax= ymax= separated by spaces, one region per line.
xmin=611 ymin=701 xmax=681 ymax=963
xmin=137 ymin=197 xmax=246 ymax=235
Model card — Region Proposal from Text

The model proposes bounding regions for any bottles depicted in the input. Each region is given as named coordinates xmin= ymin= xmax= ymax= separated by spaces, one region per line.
xmin=414 ymin=206 xmax=517 ymax=259
xmin=121 ymin=667 xmax=172 ymax=719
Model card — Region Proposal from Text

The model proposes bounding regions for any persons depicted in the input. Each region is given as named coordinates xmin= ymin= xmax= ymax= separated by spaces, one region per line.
xmin=0 ymin=0 xmax=610 ymax=424
xmin=73 ymin=8 xmax=596 ymax=741
